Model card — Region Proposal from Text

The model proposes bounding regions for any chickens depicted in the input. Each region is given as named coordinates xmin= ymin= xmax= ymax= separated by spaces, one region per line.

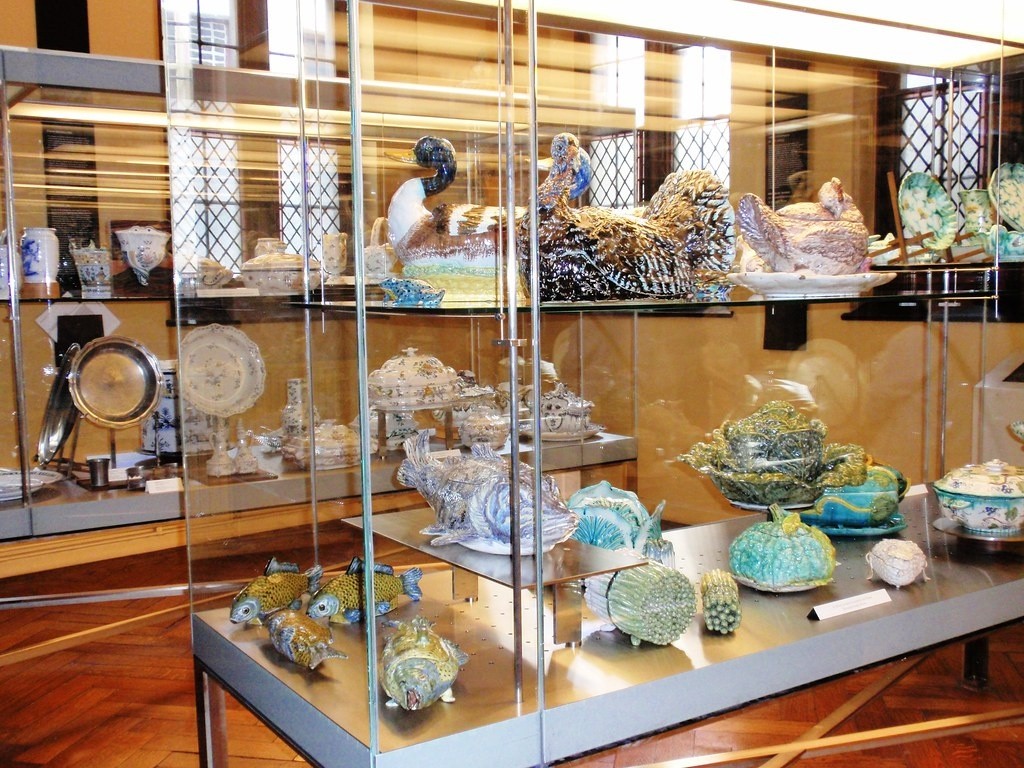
xmin=738 ymin=177 xmax=872 ymax=276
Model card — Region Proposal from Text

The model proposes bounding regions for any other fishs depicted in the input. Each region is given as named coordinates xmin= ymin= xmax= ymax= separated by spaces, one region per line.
xmin=229 ymin=556 xmax=323 ymax=623
xmin=306 ymin=557 xmax=423 ymax=619
xmin=378 ymin=615 xmax=470 ymax=710
xmin=265 ymin=608 xmax=348 ymax=669
xmin=396 ymin=429 xmax=580 ymax=556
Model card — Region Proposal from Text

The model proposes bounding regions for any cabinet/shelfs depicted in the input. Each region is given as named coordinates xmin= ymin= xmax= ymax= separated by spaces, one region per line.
xmin=158 ymin=0 xmax=1024 ymax=768
xmin=0 ymin=51 xmax=637 ymax=542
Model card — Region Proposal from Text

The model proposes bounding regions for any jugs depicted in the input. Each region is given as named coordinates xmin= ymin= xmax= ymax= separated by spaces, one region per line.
xmin=362 ymin=216 xmax=399 ymax=276
xmin=206 ymin=415 xmax=257 ymax=476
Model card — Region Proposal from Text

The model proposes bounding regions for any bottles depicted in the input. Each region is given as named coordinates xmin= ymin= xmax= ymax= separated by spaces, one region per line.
xmin=20 ymin=227 xmax=59 ymax=283
xmin=281 ymin=378 xmax=319 ymax=437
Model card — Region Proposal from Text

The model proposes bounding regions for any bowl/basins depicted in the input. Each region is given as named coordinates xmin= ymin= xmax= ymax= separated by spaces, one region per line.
xmin=931 ymin=459 xmax=1024 ymax=537
xmin=235 ymin=242 xmax=322 ymax=295
xmin=932 ymin=243 xmax=989 ymax=264
xmin=867 ymin=246 xmax=898 ymax=267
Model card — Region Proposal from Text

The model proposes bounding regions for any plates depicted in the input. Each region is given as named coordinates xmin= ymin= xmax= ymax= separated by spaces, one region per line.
xmin=802 ymin=513 xmax=907 ymax=535
xmin=726 ymin=498 xmax=814 ymax=510
xmin=68 ymin=336 xmax=163 ymax=430
xmin=36 ymin=343 xmax=77 ymax=463
xmin=988 ymin=160 xmax=1023 ymax=232
xmin=518 ymin=424 xmax=605 ymax=441
xmin=898 ymin=171 xmax=958 ymax=252
xmin=0 ymin=468 xmax=63 ymax=502
xmin=177 ymin=323 xmax=267 ymax=419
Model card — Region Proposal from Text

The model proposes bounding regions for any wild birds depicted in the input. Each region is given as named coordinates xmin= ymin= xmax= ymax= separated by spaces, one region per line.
xmin=515 ymin=133 xmax=736 ymax=300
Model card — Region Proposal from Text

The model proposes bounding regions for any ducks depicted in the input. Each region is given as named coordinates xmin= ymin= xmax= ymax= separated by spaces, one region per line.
xmin=383 ymin=135 xmax=518 ymax=269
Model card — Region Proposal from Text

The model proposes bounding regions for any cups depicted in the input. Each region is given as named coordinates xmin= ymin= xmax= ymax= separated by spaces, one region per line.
xmin=72 ymin=249 xmax=112 ymax=299
xmin=85 ymin=458 xmax=110 ymax=487
xmin=322 ymin=233 xmax=348 ymax=275
